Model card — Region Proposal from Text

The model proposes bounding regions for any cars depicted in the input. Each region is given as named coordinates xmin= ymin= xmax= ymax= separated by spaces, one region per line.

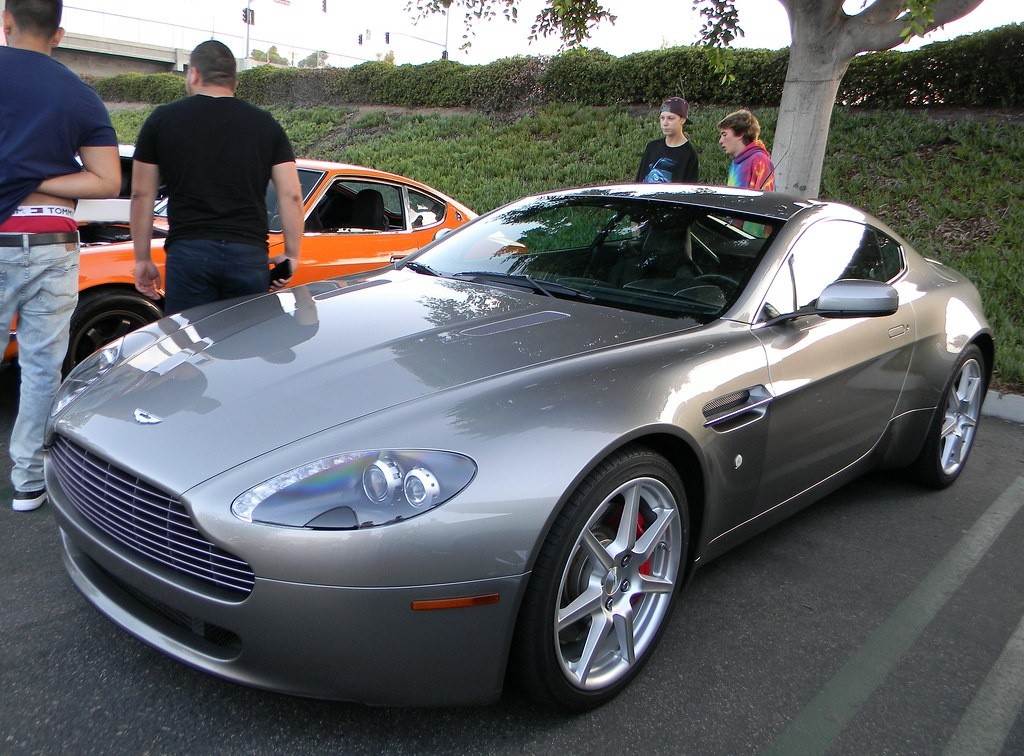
xmin=74 ymin=144 xmax=163 ymax=203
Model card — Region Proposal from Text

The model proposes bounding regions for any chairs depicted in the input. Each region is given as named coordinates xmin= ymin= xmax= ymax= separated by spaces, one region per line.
xmin=333 ymin=188 xmax=385 ymax=235
xmin=602 ymin=225 xmax=704 ymax=295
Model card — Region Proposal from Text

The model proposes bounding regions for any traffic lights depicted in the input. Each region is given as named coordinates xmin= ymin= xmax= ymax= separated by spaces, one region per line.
xmin=242 ymin=8 xmax=247 ymax=22
xmin=358 ymin=33 xmax=363 ymax=44
xmin=385 ymin=33 xmax=389 ymax=44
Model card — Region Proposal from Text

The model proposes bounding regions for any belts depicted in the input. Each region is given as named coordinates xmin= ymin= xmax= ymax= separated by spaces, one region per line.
xmin=0 ymin=231 xmax=78 ymax=248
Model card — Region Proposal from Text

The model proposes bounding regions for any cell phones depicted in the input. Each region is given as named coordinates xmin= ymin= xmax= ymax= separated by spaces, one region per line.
xmin=268 ymin=259 xmax=293 ymax=286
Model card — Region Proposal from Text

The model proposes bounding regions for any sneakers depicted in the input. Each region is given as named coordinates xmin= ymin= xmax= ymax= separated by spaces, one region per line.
xmin=11 ymin=486 xmax=48 ymax=512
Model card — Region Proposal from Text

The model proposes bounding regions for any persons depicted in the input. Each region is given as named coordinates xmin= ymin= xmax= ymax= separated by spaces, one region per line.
xmin=158 ymin=285 xmax=318 ymax=364
xmin=130 ymin=40 xmax=305 ymax=313
xmin=631 ymin=97 xmax=698 ymax=236
xmin=718 ymin=110 xmax=775 ymax=235
xmin=0 ymin=0 xmax=121 ymax=510
xmin=67 ymin=348 xmax=222 ymax=425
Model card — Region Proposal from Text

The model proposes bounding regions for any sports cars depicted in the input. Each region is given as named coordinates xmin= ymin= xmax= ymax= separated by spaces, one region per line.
xmin=41 ymin=180 xmax=995 ymax=712
xmin=2 ymin=159 xmax=532 ymax=389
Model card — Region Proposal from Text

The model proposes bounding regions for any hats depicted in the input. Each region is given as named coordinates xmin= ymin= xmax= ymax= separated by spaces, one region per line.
xmin=659 ymin=97 xmax=694 ymax=125
